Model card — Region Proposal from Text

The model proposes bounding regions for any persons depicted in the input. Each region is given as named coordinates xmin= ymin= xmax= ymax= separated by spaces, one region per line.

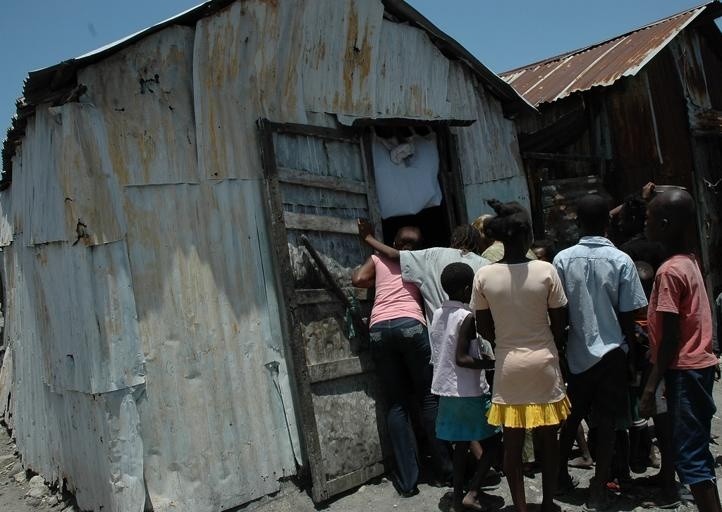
xmin=350 ymin=179 xmax=722 ymax=512
